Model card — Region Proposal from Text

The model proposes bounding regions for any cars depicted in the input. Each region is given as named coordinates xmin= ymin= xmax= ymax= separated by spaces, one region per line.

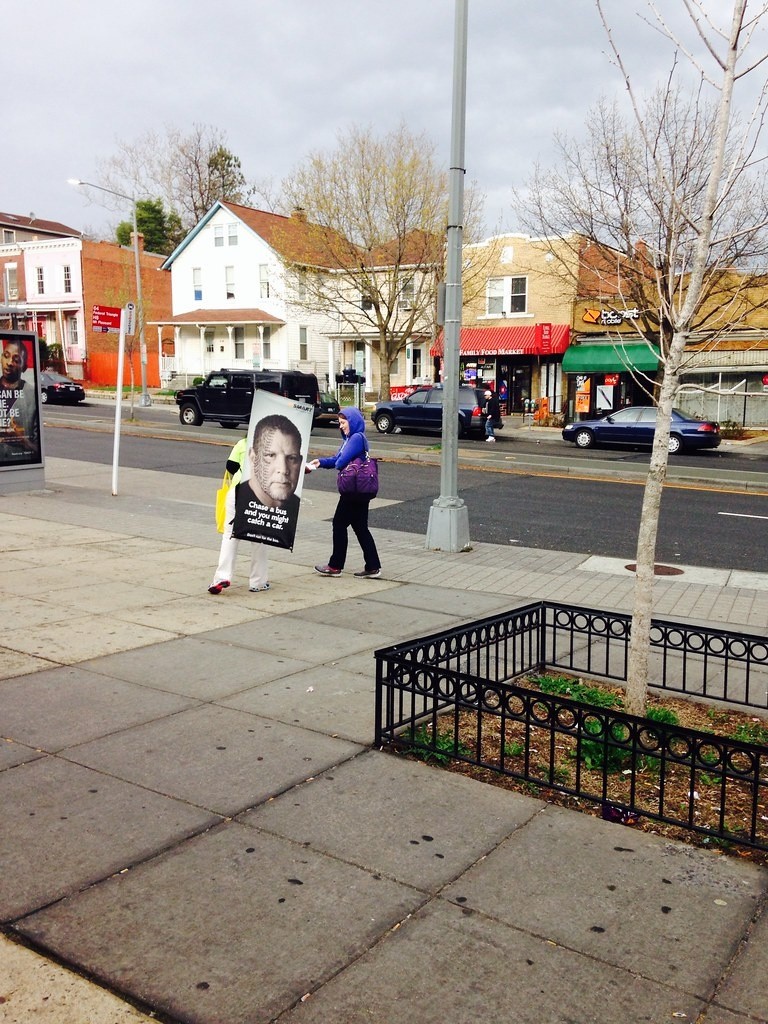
xmin=40 ymin=371 xmax=85 ymax=404
xmin=314 ymin=390 xmax=341 ymax=426
xmin=562 ymin=405 xmax=722 ymax=455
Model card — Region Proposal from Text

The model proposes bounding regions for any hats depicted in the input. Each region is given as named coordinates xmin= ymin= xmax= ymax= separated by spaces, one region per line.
xmin=484 ymin=390 xmax=492 ymax=396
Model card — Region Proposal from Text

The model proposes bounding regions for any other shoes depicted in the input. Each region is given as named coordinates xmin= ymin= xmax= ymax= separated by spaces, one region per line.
xmin=486 ymin=436 xmax=496 ymax=442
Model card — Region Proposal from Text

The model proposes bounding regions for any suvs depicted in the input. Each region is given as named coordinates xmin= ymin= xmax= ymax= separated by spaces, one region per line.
xmin=172 ymin=368 xmax=322 ymax=434
xmin=370 ymin=382 xmax=504 ymax=441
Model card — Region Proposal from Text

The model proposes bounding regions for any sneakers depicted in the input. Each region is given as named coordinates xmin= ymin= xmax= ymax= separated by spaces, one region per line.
xmin=208 ymin=579 xmax=230 ymax=595
xmin=314 ymin=565 xmax=342 ymax=577
xmin=250 ymin=582 xmax=270 ymax=592
xmin=353 ymin=567 xmax=381 ymax=579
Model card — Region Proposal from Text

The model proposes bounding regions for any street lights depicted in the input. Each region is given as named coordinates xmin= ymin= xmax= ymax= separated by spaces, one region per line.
xmin=69 ymin=177 xmax=148 ymax=409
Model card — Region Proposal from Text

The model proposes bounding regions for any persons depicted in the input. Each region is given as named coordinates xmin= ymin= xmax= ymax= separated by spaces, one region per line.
xmin=231 ymin=414 xmax=303 ymax=551
xmin=310 ymin=406 xmax=382 ymax=578
xmin=206 ymin=431 xmax=272 ymax=594
xmin=483 ymin=390 xmax=500 ymax=443
xmin=0 ymin=340 xmax=38 ymax=461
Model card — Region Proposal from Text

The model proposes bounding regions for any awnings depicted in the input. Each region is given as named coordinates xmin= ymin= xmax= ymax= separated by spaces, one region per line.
xmin=429 ymin=324 xmax=570 ymax=357
xmin=561 ymin=344 xmax=660 ymax=372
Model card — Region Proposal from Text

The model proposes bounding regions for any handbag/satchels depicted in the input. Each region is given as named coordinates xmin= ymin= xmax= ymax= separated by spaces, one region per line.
xmin=215 ymin=469 xmax=232 ymax=534
xmin=337 ymin=433 xmax=379 ymax=499
